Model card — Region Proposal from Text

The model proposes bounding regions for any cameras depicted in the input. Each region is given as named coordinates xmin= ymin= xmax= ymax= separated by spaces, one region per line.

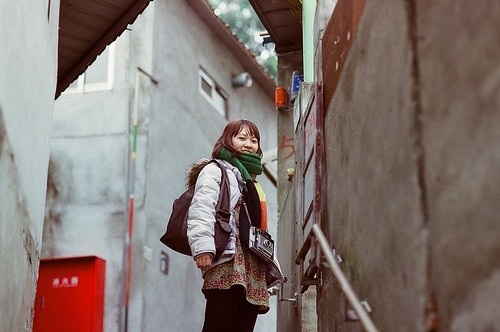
xmin=247 ymin=227 xmax=275 ymax=262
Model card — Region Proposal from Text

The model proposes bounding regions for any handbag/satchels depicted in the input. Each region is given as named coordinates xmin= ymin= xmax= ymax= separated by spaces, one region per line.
xmin=160 ymin=159 xmax=231 ymax=261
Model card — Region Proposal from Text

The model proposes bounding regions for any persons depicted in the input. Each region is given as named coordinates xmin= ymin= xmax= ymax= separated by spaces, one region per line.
xmin=187 ymin=119 xmax=285 ymax=332
